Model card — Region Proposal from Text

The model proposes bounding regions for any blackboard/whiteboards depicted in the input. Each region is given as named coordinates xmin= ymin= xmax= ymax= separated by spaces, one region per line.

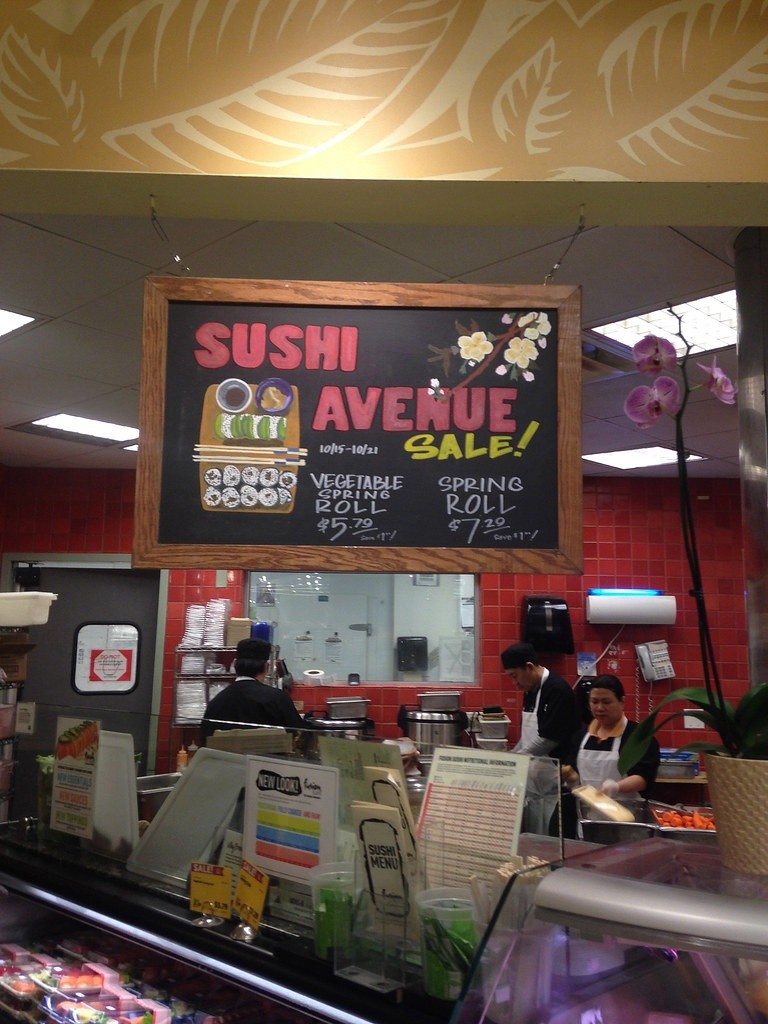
xmin=131 ymin=275 xmax=584 ymax=573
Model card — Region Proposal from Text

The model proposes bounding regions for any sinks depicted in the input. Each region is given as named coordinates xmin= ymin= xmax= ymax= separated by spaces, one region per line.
xmin=136 ymin=771 xmax=183 ymax=823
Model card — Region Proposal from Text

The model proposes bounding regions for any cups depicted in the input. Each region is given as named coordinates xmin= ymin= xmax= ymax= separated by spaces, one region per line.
xmin=310 ymin=861 xmax=366 ymax=961
xmin=414 ymin=888 xmax=475 ymax=1000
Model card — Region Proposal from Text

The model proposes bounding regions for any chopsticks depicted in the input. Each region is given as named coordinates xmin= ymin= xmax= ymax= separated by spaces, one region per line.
xmin=190 ymin=455 xmax=307 ymax=466
xmin=468 ymin=853 xmax=559 ymax=933
xmin=192 ymin=444 xmax=308 ymax=456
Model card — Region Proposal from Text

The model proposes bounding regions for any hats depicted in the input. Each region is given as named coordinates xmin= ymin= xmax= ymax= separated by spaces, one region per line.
xmin=501 ymin=643 xmax=537 ymax=669
xmin=237 ymin=638 xmax=271 ymax=661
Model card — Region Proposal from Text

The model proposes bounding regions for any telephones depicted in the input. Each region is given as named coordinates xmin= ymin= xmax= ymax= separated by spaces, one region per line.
xmin=635 ymin=639 xmax=676 ymax=683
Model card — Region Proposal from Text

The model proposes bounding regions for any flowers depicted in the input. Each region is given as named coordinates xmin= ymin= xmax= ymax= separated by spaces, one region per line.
xmin=616 ymin=302 xmax=768 ymax=777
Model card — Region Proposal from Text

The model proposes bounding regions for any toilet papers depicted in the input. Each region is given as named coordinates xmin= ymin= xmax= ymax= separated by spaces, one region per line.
xmin=303 ymin=669 xmax=325 ymax=685
xmin=585 ymin=595 xmax=677 ymax=625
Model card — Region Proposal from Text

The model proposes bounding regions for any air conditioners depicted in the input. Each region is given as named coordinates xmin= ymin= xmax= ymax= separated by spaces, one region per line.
xmin=586 ymin=596 xmax=677 ymax=625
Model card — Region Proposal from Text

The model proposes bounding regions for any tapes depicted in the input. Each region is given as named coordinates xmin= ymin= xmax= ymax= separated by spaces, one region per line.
xmin=303 ymin=669 xmax=337 ymax=686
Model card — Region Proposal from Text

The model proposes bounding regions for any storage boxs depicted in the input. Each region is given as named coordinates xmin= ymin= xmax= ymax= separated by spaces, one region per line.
xmin=479 ymin=714 xmax=512 ymax=738
xmin=419 ymin=690 xmax=461 ymax=711
xmin=474 ymin=733 xmax=509 ymax=749
xmin=324 ymin=695 xmax=372 ymax=719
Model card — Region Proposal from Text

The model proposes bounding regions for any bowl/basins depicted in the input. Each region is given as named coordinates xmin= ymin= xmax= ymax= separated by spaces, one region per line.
xmin=255 ymin=377 xmax=293 ymax=414
xmin=216 ymin=378 xmax=252 ymax=413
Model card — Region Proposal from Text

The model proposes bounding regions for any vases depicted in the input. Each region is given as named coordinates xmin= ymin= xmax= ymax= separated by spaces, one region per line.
xmin=704 ymin=752 xmax=768 ymax=876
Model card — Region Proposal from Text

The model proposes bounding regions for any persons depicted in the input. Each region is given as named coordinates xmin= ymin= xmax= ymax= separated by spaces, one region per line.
xmin=500 ymin=643 xmax=583 ymax=835
xmin=199 ymin=638 xmax=304 ymax=746
xmin=578 ymin=674 xmax=662 ymax=842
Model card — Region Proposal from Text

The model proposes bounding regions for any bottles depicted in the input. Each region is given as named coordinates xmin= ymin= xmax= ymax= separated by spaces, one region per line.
xmin=177 ymin=745 xmax=188 ymax=768
xmin=188 ymin=740 xmax=198 ymax=759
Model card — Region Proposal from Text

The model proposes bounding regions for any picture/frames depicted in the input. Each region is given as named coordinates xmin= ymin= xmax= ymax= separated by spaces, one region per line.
xmin=413 ymin=573 xmax=440 ymax=587
